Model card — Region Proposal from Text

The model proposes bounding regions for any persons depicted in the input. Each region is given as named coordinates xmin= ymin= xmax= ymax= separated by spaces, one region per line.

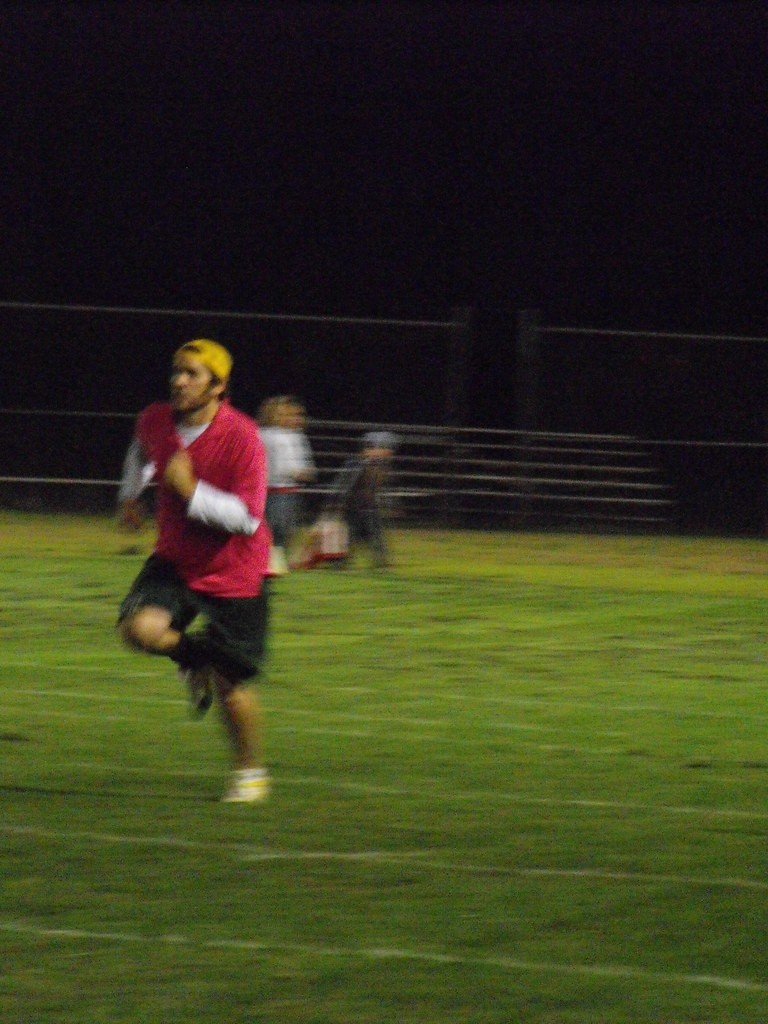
xmin=321 ymin=430 xmax=402 ymax=568
xmin=113 ymin=338 xmax=273 ymax=806
xmin=252 ymin=395 xmax=316 ymax=578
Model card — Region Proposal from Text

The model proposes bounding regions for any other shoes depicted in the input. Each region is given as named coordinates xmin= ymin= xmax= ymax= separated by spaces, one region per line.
xmin=179 ymin=655 xmax=214 ymax=725
xmin=219 ymin=768 xmax=269 ymax=806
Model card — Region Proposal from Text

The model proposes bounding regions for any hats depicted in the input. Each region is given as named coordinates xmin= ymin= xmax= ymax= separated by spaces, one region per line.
xmin=173 ymin=340 xmax=235 ymax=382
xmin=366 ymin=431 xmax=404 ymax=449
